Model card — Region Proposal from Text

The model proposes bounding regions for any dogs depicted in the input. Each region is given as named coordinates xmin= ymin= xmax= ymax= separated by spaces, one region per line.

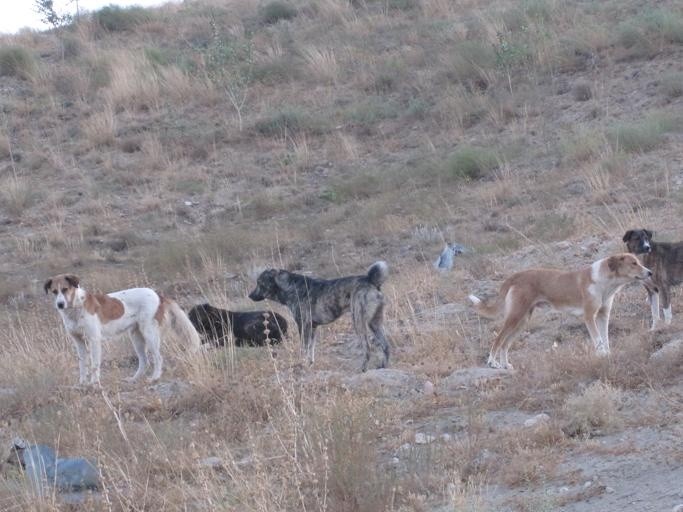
xmin=248 ymin=260 xmax=390 ymax=375
xmin=623 ymin=229 xmax=683 ymax=331
xmin=188 ymin=304 xmax=286 ymax=348
xmin=44 ymin=274 xmax=201 ymax=388
xmin=467 ymin=252 xmax=652 ymax=372
xmin=6 ymin=436 xmax=103 ymax=504
